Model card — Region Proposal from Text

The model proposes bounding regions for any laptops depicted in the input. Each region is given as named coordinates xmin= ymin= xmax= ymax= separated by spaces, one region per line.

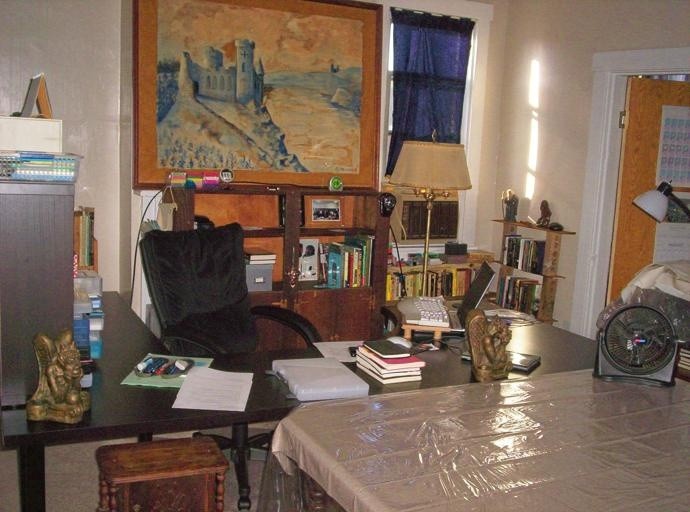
xmin=452 ymin=261 xmax=496 ymax=331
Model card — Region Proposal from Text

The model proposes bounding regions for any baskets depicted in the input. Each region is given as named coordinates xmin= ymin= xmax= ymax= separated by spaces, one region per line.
xmin=0 ymin=152 xmax=85 ymax=182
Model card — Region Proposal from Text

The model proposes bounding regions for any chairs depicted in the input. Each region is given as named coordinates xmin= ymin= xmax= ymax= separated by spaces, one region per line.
xmin=139 ymin=221 xmax=325 ymax=459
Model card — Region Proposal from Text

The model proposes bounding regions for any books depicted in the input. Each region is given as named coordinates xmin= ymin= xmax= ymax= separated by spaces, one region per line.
xmin=676 ymin=348 xmax=690 ymax=371
xmin=329 ymin=239 xmax=366 ymax=289
xmin=244 ymin=246 xmax=277 ymax=265
xmin=384 ymin=267 xmax=475 ymax=300
xmin=1 ymin=150 xmax=80 ymax=185
xmin=355 ymin=340 xmax=426 ymax=385
xmin=72 ymin=208 xmax=94 ymax=267
xmin=461 ymin=350 xmax=541 ymax=374
xmin=496 ymin=235 xmax=545 ymax=315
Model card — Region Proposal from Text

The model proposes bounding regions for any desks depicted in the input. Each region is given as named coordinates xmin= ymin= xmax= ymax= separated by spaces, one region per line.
xmin=269 ymin=367 xmax=689 ymax=512
xmin=0 ymin=289 xmax=598 ymax=512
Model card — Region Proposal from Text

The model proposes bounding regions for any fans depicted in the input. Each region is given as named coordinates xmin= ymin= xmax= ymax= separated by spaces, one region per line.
xmin=596 ymin=302 xmax=678 ymax=388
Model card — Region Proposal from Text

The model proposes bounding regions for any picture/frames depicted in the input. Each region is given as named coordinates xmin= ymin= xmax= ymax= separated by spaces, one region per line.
xmin=132 ymin=0 xmax=384 ymax=192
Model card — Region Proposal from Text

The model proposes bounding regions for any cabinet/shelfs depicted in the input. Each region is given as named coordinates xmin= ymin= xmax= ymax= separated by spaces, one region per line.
xmin=387 ymin=263 xmax=484 ymax=301
xmin=0 ymin=181 xmax=75 ymax=409
xmin=162 ymin=187 xmax=389 ymax=349
xmin=492 ymin=218 xmax=576 ymax=325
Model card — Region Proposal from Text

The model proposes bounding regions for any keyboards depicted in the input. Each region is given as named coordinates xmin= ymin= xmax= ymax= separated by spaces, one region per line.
xmin=397 ymin=296 xmax=449 ymax=326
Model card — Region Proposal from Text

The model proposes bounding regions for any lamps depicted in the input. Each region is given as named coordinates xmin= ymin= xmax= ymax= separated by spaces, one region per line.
xmin=388 ymin=140 xmax=473 ymax=297
xmin=632 ymin=181 xmax=690 ymax=223
